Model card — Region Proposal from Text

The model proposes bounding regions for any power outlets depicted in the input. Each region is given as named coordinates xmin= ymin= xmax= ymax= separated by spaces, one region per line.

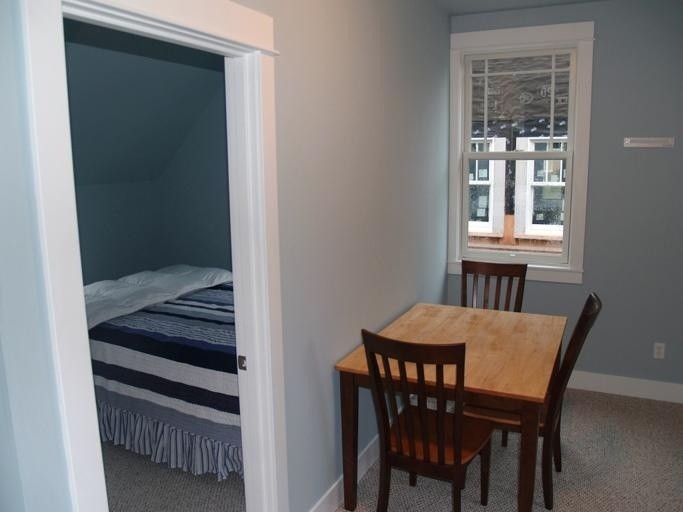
xmin=652 ymin=340 xmax=668 ymax=361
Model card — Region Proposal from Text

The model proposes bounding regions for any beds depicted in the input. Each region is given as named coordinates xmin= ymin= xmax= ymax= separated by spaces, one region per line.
xmin=77 ymin=263 xmax=245 ymax=471
